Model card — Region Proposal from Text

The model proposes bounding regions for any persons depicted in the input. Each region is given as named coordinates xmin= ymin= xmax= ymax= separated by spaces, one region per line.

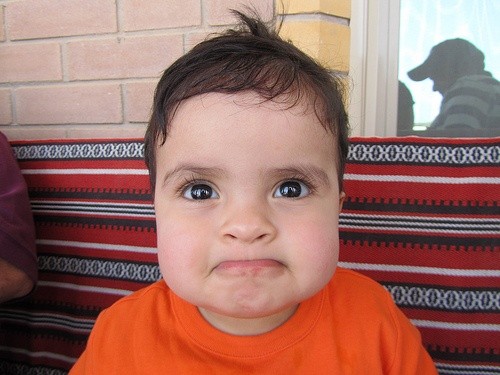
xmin=408 ymin=37 xmax=500 ymax=131
xmin=69 ymin=7 xmax=438 ymax=375
xmin=0 ymin=130 xmax=40 ymax=303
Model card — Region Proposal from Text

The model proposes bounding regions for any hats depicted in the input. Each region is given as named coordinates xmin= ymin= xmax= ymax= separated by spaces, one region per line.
xmin=407 ymin=38 xmax=485 ymax=81
